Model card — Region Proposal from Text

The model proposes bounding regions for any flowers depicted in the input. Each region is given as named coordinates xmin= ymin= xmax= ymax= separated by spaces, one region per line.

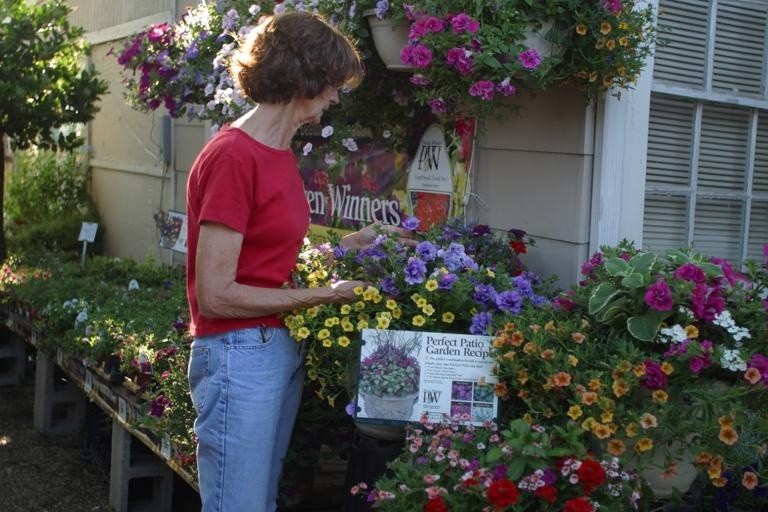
xmin=0 ymin=1 xmax=767 ymax=512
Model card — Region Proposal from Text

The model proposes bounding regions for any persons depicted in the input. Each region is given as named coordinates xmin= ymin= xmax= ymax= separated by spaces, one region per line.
xmin=183 ymin=7 xmax=413 ymax=512
